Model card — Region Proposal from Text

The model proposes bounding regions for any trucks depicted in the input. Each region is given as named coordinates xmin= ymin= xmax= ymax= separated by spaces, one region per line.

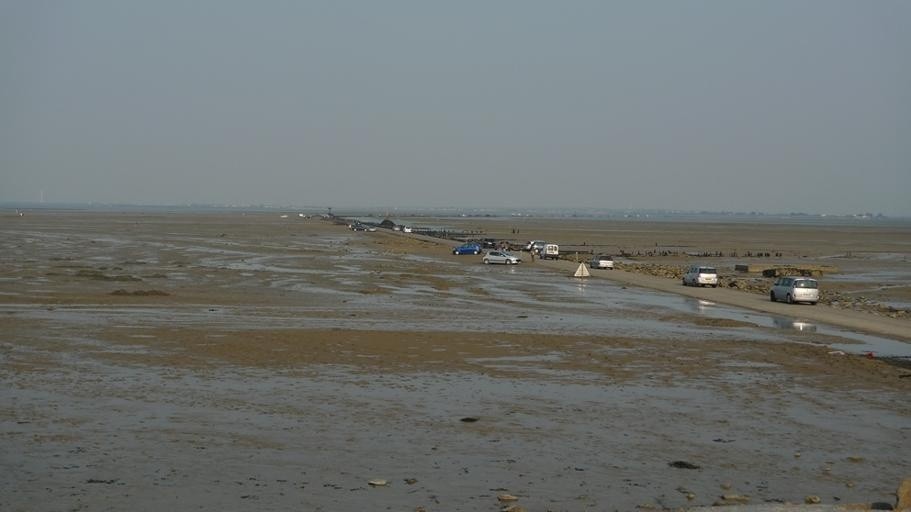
xmin=543 ymin=244 xmax=559 ymax=260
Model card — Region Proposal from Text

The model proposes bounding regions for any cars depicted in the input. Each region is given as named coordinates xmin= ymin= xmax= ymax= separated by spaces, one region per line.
xmin=590 ymin=256 xmax=613 ymax=270
xmin=392 ymin=226 xmax=400 ymax=230
xmin=368 ymin=227 xmax=377 ymax=232
xmin=453 ymin=243 xmax=481 ymax=254
xmin=482 ymin=250 xmax=521 ymax=264
xmin=349 ymin=224 xmax=367 ymax=231
xmin=403 ymin=226 xmax=413 ymax=233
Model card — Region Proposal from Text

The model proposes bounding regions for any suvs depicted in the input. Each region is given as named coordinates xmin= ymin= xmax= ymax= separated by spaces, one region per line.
xmin=531 ymin=240 xmax=545 ymax=254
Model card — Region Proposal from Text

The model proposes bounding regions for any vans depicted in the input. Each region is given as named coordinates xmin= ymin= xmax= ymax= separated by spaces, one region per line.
xmin=769 ymin=275 xmax=819 ymax=305
xmin=683 ymin=266 xmax=717 ymax=288
